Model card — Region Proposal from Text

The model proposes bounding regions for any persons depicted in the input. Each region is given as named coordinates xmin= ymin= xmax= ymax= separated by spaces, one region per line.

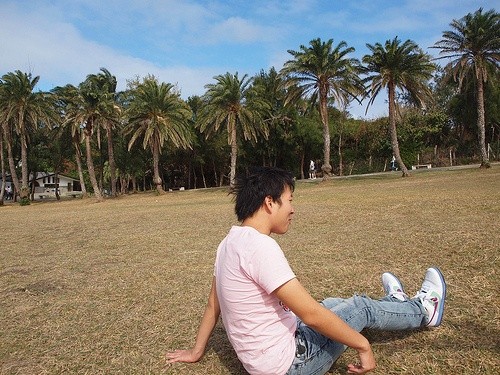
xmin=390 ymin=152 xmax=398 ymax=171
xmin=6 ymin=186 xmax=11 ymax=200
xmin=308 ymin=160 xmax=316 ymax=179
xmin=165 ymin=166 xmax=446 ymax=375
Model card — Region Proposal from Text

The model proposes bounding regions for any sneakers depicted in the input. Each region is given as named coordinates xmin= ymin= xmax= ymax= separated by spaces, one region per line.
xmin=411 ymin=267 xmax=446 ymax=327
xmin=381 ymin=272 xmax=410 ymax=302
